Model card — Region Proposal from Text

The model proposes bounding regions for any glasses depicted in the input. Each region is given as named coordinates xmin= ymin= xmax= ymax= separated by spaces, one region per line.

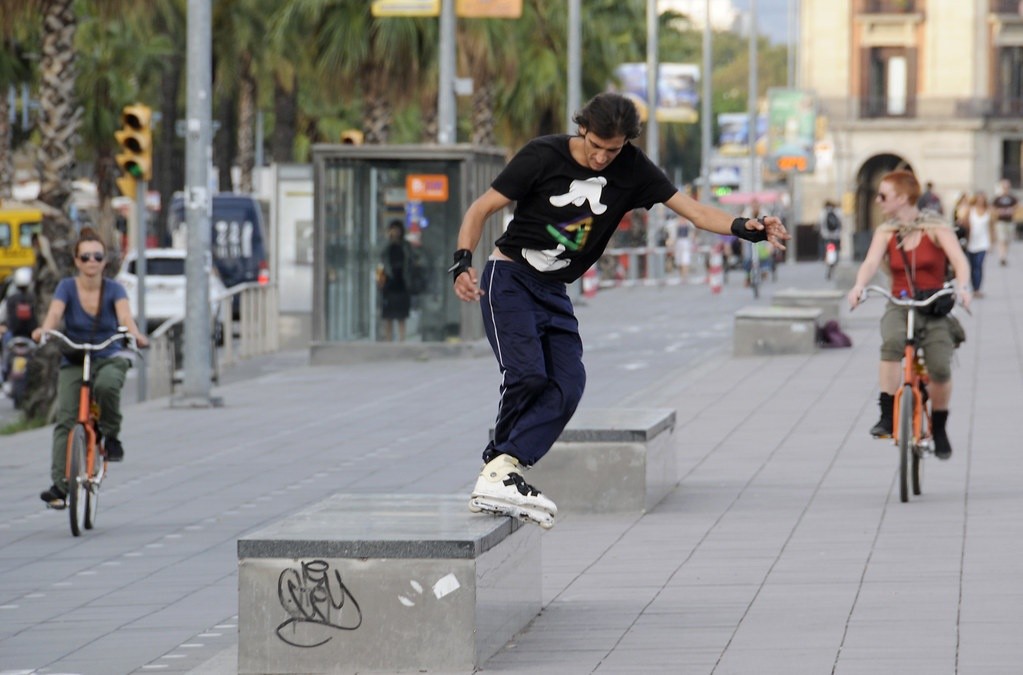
xmin=78 ymin=252 xmax=105 ymax=262
xmin=878 ymin=193 xmax=900 ymax=201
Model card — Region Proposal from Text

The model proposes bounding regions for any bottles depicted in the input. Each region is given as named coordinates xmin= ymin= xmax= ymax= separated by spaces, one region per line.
xmin=900 ymin=290 xmax=924 ymax=330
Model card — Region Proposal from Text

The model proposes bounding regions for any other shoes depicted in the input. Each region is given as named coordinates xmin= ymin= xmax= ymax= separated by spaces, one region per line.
xmin=105 ymin=438 xmax=123 ymax=461
xmin=41 ymin=485 xmax=67 ymax=508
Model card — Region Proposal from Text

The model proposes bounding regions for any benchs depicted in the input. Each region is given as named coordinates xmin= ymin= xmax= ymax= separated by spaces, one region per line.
xmin=732 ymin=308 xmax=822 ymax=356
xmin=770 ymin=290 xmax=846 ymax=325
xmin=489 ymin=409 xmax=676 ymax=514
xmin=235 ymin=494 xmax=543 ymax=675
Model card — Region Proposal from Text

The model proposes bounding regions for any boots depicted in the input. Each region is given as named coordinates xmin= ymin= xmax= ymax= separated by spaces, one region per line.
xmin=870 ymin=393 xmax=895 ymax=435
xmin=932 ymin=411 xmax=952 ymax=459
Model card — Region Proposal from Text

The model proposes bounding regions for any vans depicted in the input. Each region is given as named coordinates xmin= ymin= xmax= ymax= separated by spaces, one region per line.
xmin=164 ymin=191 xmax=272 ymax=319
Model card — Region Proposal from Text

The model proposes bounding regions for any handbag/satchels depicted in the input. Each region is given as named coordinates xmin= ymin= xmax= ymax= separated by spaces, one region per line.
xmin=917 ymin=288 xmax=955 ymax=317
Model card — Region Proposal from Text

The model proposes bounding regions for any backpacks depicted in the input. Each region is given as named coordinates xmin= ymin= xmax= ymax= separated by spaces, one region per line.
xmin=392 ymin=240 xmax=428 ymax=294
xmin=8 ymin=294 xmax=37 ymax=337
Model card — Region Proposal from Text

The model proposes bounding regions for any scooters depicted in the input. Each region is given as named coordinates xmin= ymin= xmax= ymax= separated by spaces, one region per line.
xmin=3 ymin=322 xmax=47 ymax=420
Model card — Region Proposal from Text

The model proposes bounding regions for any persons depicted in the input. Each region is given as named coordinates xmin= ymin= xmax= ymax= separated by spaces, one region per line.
xmin=375 ymin=219 xmax=419 ymax=342
xmin=822 ymin=200 xmax=845 ymax=283
xmin=849 ymin=164 xmax=975 ymax=461
xmin=34 ymin=224 xmax=150 ymax=511
xmin=0 ymin=267 xmax=37 ymax=390
xmin=739 ymin=198 xmax=771 ymax=295
xmin=456 ymin=91 xmax=792 ymax=531
xmin=917 ymin=174 xmax=1023 ymax=287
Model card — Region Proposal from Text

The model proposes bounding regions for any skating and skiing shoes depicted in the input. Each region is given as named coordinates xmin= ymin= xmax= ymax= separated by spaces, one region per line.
xmin=468 ymin=454 xmax=557 ymax=530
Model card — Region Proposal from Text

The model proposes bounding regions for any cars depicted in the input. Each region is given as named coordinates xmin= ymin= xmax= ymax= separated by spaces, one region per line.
xmin=112 ymin=246 xmax=234 ymax=350
xmin=0 ymin=190 xmax=163 ymax=299
xmin=598 ymin=189 xmax=786 ymax=275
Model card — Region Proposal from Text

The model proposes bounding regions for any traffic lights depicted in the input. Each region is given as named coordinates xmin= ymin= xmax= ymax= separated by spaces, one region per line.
xmin=340 ymin=128 xmax=363 ymax=146
xmin=114 ymin=130 xmax=137 ymax=199
xmin=122 ymin=102 xmax=153 ymax=183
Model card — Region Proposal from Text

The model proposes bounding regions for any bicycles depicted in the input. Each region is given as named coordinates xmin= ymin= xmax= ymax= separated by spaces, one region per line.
xmin=40 ymin=325 xmax=150 ymax=537
xmin=850 ymin=276 xmax=974 ymax=503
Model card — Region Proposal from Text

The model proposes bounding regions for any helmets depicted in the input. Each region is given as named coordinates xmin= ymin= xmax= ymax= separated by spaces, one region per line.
xmin=14 ymin=266 xmax=33 ymax=286
